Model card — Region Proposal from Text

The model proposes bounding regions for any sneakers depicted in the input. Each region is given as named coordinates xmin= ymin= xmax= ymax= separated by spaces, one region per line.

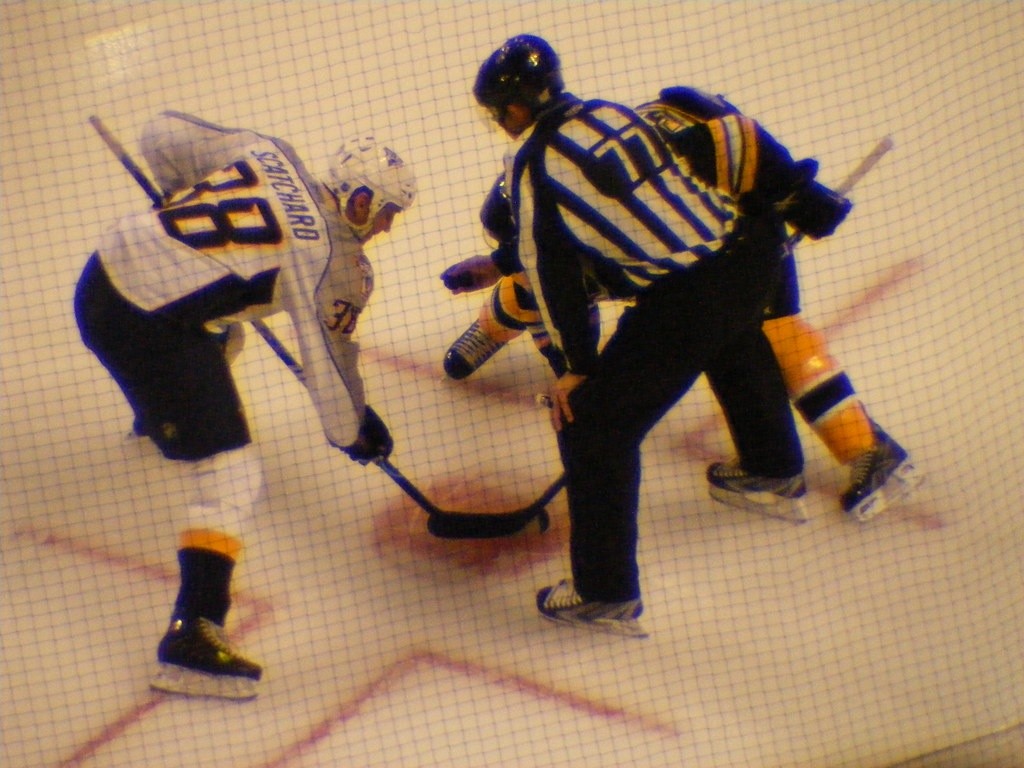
xmin=844 ymin=419 xmax=925 ymax=523
xmin=125 ymin=419 xmax=143 ymax=439
xmin=707 ymin=459 xmax=810 ymax=522
xmin=149 ymin=608 xmax=264 ymax=700
xmin=440 ymin=320 xmax=507 ymax=381
xmin=537 ymin=579 xmax=643 ymax=636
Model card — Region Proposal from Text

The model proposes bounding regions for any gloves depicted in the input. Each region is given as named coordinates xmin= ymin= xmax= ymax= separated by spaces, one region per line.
xmin=340 ymin=404 xmax=393 ymax=467
xmin=782 ymin=166 xmax=853 ymax=239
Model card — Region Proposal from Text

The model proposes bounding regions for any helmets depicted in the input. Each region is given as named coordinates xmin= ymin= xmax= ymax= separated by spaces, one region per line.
xmin=335 ymin=136 xmax=416 ymax=210
xmin=481 ymin=172 xmax=517 ymax=245
xmin=474 ymin=34 xmax=564 ymax=108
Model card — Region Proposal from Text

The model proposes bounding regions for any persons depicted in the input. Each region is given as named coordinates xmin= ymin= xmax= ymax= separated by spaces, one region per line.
xmin=439 ymin=33 xmax=910 ymax=622
xmin=73 ymin=110 xmax=417 ymax=681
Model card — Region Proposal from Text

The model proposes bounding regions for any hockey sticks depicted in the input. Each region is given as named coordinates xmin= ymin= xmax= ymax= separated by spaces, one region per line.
xmin=425 ymin=131 xmax=896 ymax=541
xmin=90 ymin=112 xmax=550 ymax=541
